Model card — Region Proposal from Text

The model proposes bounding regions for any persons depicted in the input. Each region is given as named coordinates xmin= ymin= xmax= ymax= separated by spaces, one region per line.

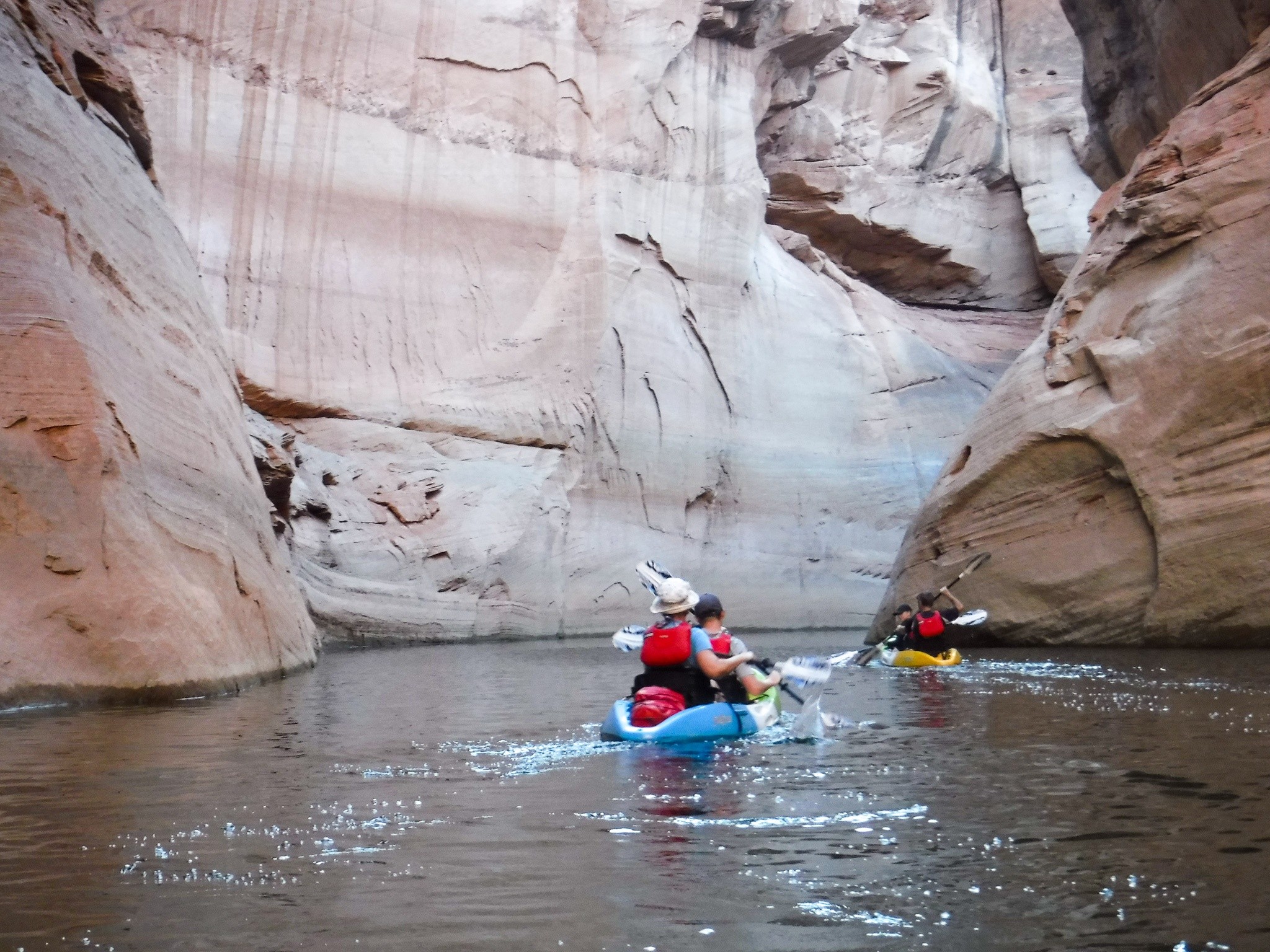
xmin=631 ymin=577 xmax=784 ymax=710
xmin=886 ymin=586 xmax=964 ymax=658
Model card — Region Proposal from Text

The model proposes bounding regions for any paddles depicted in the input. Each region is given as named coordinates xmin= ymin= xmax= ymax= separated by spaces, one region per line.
xmin=856 ymin=551 xmax=991 ymax=667
xmin=850 ymin=609 xmax=988 ymax=668
xmin=635 ymin=556 xmax=832 ymax=728
xmin=611 ymin=623 xmax=831 ymax=689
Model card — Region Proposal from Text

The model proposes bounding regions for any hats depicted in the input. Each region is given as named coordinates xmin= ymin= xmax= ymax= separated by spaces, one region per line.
xmin=694 ymin=593 xmax=723 ymax=617
xmin=650 ymin=577 xmax=700 ymax=615
xmin=892 ymin=604 xmax=912 ymax=616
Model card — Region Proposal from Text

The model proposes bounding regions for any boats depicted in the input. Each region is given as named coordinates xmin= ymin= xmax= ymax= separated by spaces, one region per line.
xmin=878 ymin=646 xmax=963 ymax=668
xmin=601 ymin=667 xmax=781 ymax=743
